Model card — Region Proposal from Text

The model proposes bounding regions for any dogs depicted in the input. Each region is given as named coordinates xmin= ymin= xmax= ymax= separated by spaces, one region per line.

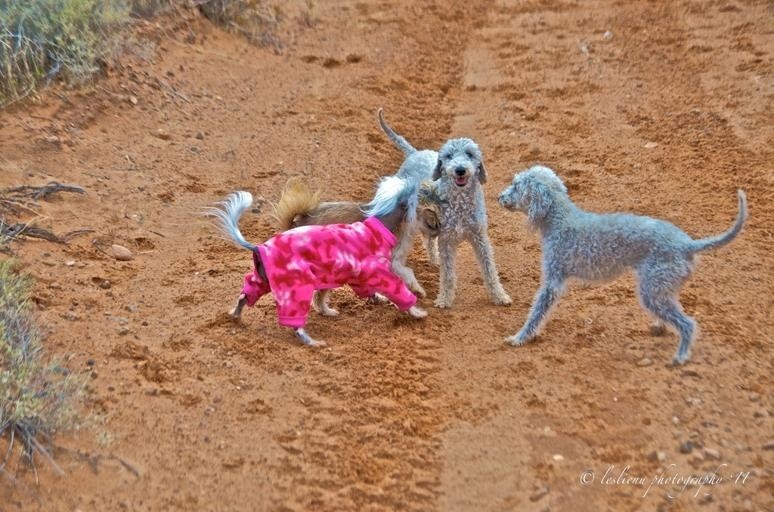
xmin=203 ymin=177 xmax=428 ymax=351
xmin=267 ymin=177 xmax=448 ymax=318
xmin=495 ymin=165 xmax=747 ymax=367
xmin=377 ymin=107 xmax=514 ymax=309
